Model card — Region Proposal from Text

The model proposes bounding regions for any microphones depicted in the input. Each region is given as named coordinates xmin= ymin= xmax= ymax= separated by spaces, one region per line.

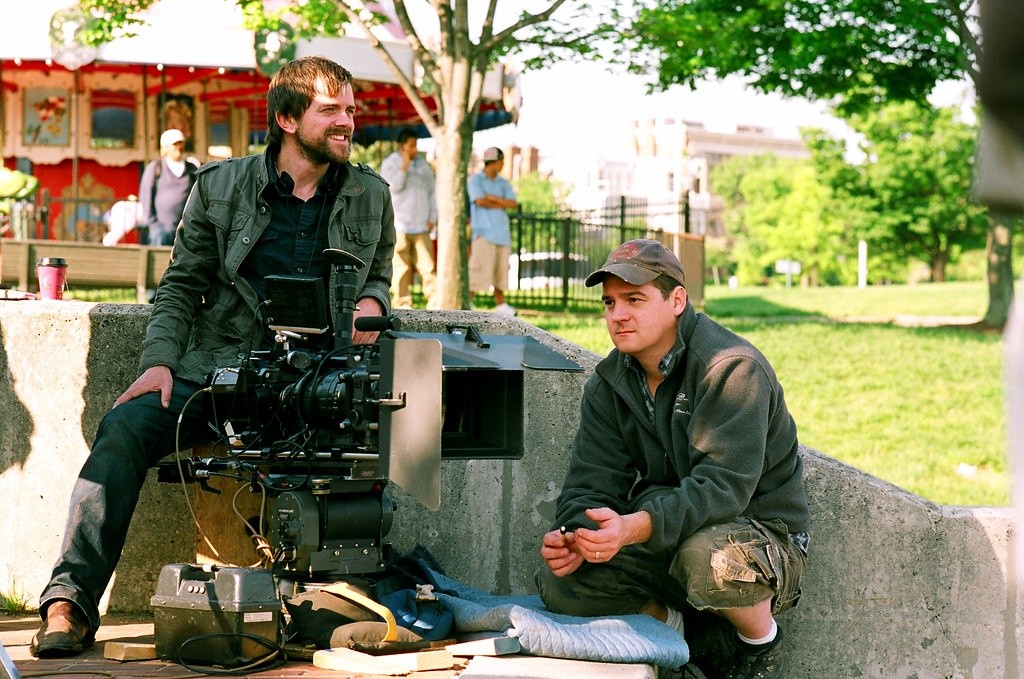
xmin=354 ymin=316 xmax=402 ymax=331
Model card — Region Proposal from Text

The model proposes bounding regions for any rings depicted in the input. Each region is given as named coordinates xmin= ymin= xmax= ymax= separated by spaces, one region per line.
xmin=595 ymin=551 xmax=600 ymax=559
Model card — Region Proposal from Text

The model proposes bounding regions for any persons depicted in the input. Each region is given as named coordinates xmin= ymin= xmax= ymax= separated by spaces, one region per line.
xmin=383 ymin=128 xmax=438 ymax=312
xmin=29 ymin=58 xmax=396 ymax=659
xmin=466 ymin=147 xmax=520 ymax=318
xmin=540 ymin=238 xmax=809 ymax=679
xmin=137 ymin=129 xmax=198 ymax=246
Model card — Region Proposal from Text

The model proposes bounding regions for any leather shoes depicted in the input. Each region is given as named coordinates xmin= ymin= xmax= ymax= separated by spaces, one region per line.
xmin=30 ymin=600 xmax=89 ymax=655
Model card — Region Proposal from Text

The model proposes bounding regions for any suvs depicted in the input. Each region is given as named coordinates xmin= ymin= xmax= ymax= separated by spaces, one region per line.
xmin=507 ymin=252 xmax=591 ymax=292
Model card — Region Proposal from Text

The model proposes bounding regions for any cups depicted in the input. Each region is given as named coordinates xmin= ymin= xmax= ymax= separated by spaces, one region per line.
xmin=36 ymin=258 xmax=69 ymax=300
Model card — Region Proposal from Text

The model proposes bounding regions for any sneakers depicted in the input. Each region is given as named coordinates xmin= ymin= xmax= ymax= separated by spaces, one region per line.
xmin=666 ymin=615 xmax=719 ymax=679
xmin=719 ymin=627 xmax=785 ymax=679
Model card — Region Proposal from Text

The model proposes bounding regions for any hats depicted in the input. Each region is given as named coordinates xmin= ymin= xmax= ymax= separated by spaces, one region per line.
xmin=484 ymin=147 xmax=503 ymax=162
xmin=585 ymin=238 xmax=685 ymax=290
xmin=161 ymin=129 xmax=185 ymax=146
